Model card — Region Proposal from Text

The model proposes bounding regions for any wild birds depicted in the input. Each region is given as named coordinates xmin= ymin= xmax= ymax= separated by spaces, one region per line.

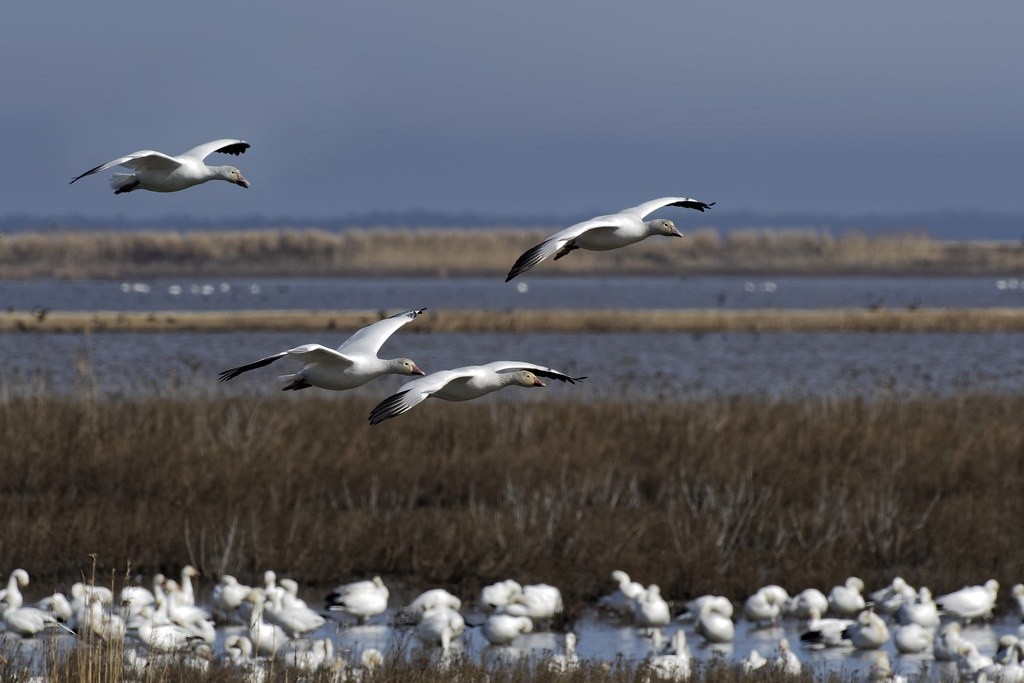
xmin=504 ymin=193 xmax=715 ymax=284
xmin=65 ymin=137 xmax=251 ymax=198
xmin=0 ymin=562 xmax=1024 ymax=683
xmin=216 ymin=308 xmax=426 ymax=389
xmin=368 ymin=360 xmax=585 ymax=430
